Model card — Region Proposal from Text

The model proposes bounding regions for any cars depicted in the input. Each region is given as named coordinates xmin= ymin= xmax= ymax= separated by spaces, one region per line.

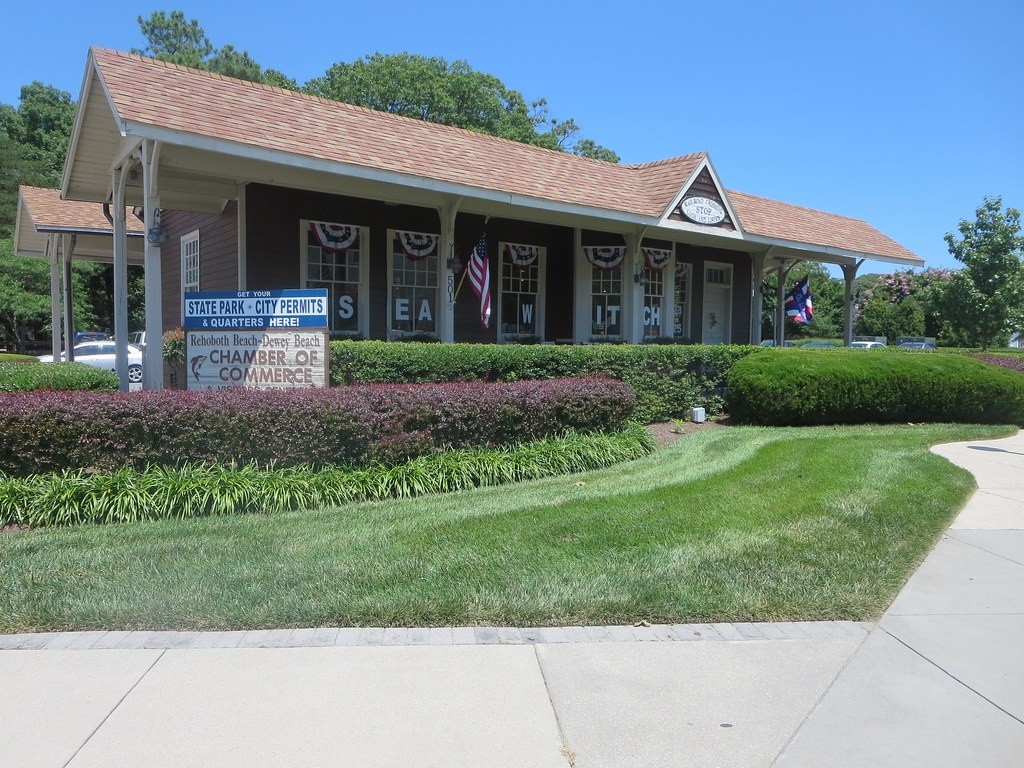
xmin=897 ymin=343 xmax=937 ymax=349
xmin=36 ymin=340 xmax=143 ymax=383
xmin=760 ymin=340 xmax=795 ymax=347
xmin=800 ymin=343 xmax=837 ymax=348
xmin=850 ymin=342 xmax=887 ymax=349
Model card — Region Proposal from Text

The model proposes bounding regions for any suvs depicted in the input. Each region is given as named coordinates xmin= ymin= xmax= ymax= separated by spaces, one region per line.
xmin=128 ymin=331 xmax=146 ymax=349
xmin=74 ymin=331 xmax=108 ymax=347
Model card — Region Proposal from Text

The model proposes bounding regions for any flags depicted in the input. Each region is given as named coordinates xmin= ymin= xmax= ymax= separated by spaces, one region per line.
xmin=465 ymin=226 xmax=492 ymax=331
xmin=785 ymin=272 xmax=814 ymax=326
